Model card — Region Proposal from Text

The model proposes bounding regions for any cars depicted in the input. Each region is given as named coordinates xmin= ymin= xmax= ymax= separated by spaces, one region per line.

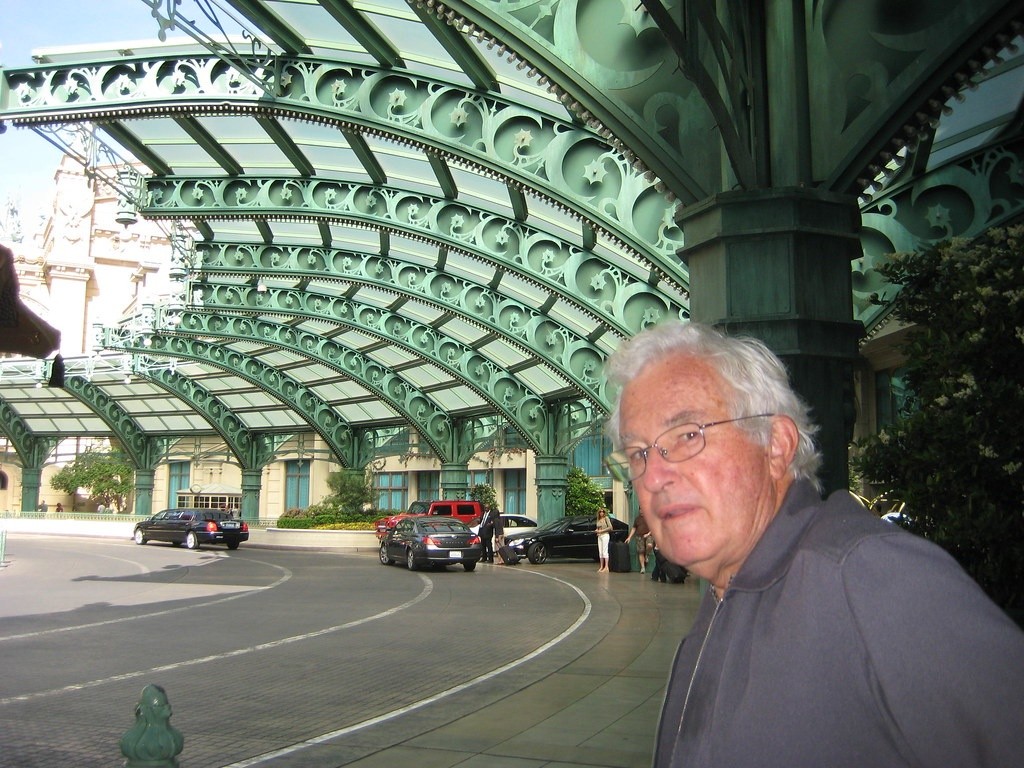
xmin=504 ymin=515 xmax=629 ymax=565
xmin=467 ymin=513 xmax=537 ymax=555
xmin=133 ymin=507 xmax=250 ymax=550
xmin=379 ymin=515 xmax=482 ymax=571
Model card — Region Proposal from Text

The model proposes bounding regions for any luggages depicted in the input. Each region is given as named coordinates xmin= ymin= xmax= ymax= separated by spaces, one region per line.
xmin=495 ymin=540 xmax=518 ymax=566
xmin=658 ymin=555 xmax=687 ymax=584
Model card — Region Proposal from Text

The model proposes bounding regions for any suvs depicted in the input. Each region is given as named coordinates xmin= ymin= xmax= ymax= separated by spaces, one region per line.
xmin=374 ymin=499 xmax=482 ymax=540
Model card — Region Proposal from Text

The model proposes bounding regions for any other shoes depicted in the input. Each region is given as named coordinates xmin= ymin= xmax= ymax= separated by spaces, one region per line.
xmin=485 ymin=560 xmax=493 ymax=563
xmin=651 ymin=577 xmax=658 ymax=581
xmin=597 ymin=568 xmax=609 ymax=573
xmin=479 ymin=560 xmax=485 ymax=563
xmin=494 ymin=561 xmax=504 ymax=565
xmin=640 ymin=568 xmax=645 ymax=574
xmin=660 ymin=577 xmax=666 ymax=583
xmin=645 ymin=554 xmax=649 ymax=563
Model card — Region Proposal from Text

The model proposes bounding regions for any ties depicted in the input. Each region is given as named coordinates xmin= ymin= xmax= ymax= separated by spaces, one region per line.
xmin=482 ymin=512 xmax=487 ymax=527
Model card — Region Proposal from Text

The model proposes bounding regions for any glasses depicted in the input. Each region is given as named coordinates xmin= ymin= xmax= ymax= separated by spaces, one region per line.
xmin=600 ymin=414 xmax=777 ymax=483
xmin=599 ymin=510 xmax=605 ymax=513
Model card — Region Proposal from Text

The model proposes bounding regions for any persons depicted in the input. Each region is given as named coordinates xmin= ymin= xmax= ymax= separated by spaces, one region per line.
xmin=477 ymin=504 xmax=494 ymax=563
xmin=592 ymin=508 xmax=614 ymax=574
xmin=231 ymin=504 xmax=241 ymax=519
xmin=491 ymin=508 xmax=507 ymax=565
xmin=591 ymin=311 xmax=1023 ymax=768
xmin=55 ymin=503 xmax=64 ymax=513
xmin=623 ymin=504 xmax=655 ymax=574
xmin=97 ymin=502 xmax=106 ymax=514
xmin=37 ymin=499 xmax=49 ymax=513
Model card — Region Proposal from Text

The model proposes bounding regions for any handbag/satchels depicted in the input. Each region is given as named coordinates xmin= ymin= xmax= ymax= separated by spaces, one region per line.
xmin=605 ymin=516 xmax=613 ymax=532
